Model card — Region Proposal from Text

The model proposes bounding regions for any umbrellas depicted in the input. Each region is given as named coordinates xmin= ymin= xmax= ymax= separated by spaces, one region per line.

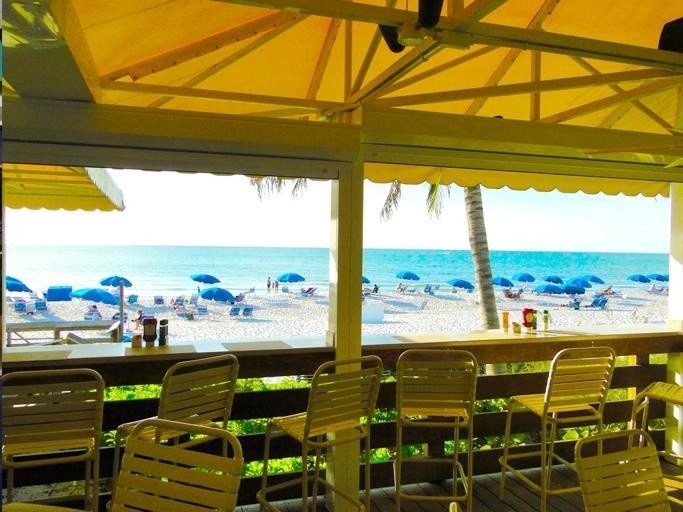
xmin=99 ymin=276 xmax=132 ymax=288
xmin=5 ymin=275 xmax=30 ymax=292
xmin=276 ymin=272 xmax=305 ymax=282
xmin=70 ymin=287 xmax=119 ymax=306
xmin=198 ymin=286 xmax=233 ymax=302
xmin=625 ymin=273 xmax=669 ymax=284
xmin=492 ymin=271 xmax=605 ymax=295
xmin=362 ymin=276 xmax=370 ymax=284
xmin=395 ymin=271 xmax=420 ymax=281
xmin=190 ymin=273 xmax=220 ymax=284
xmin=446 ymin=279 xmax=475 ymax=290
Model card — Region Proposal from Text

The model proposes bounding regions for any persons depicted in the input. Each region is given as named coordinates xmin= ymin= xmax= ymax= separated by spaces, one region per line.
xmin=275 ymin=281 xmax=279 ymax=289
xmin=131 ymin=309 xmax=144 ymax=329
xmin=267 ymin=276 xmax=272 ymax=288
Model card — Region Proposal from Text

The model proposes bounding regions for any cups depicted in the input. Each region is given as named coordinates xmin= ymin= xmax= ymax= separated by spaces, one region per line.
xmin=511 ymin=317 xmax=521 ymax=334
xmin=130 ymin=332 xmax=141 ymax=349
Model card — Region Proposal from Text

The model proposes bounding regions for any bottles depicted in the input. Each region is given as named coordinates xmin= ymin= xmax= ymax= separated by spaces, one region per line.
xmin=533 ymin=309 xmax=537 ymax=332
xmin=521 ymin=307 xmax=534 ymax=334
xmin=162 ymin=318 xmax=169 ymax=346
xmin=142 ymin=318 xmax=157 ymax=349
xmin=542 ymin=309 xmax=548 ymax=332
xmin=158 ymin=320 xmax=166 ymax=347
xmin=501 ymin=310 xmax=509 ymax=334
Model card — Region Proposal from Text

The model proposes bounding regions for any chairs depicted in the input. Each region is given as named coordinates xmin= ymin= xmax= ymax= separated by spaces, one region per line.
xmin=498 ymin=346 xmax=683 ymax=512
xmin=7 ymin=292 xmax=48 ymax=313
xmin=229 ymin=287 xmax=256 ymax=319
xmin=84 ymin=314 xmax=101 ymax=320
xmin=256 ymin=355 xmax=384 ymax=512
xmin=362 ymin=284 xmax=418 ymax=299
xmin=128 ymin=295 xmax=207 ymax=333
xmin=393 ymin=349 xmax=478 ymax=512
xmin=567 ymin=294 xmax=608 ymax=310
xmin=0 ymin=353 xmax=244 ymax=512
xmin=499 ymin=289 xmax=531 ymax=307
xmin=281 ymin=286 xmax=317 ymax=299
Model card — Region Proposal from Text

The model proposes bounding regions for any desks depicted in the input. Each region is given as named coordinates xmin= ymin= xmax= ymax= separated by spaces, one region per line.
xmin=55 ymin=320 xmax=120 ymax=342
xmin=6 ymin=321 xmax=56 ymax=347
